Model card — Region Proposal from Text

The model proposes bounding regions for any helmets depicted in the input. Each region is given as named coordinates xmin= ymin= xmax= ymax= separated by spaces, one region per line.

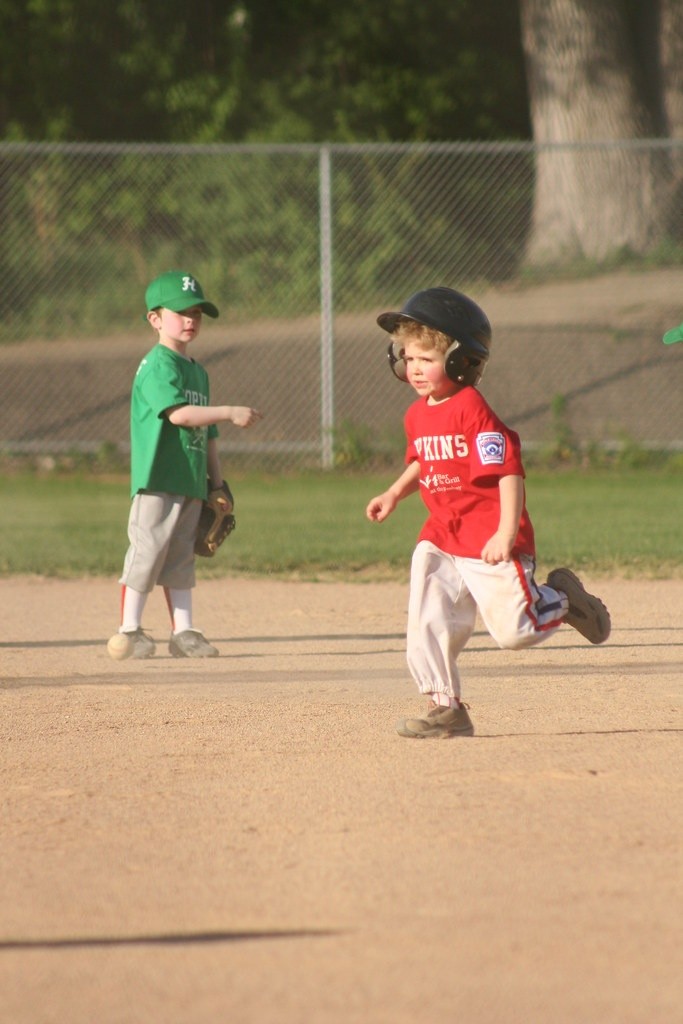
xmin=377 ymin=287 xmax=492 ymax=386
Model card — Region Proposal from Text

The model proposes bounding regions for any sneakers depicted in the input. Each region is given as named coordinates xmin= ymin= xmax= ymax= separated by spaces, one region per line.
xmin=547 ymin=568 xmax=611 ymax=644
xmin=396 ymin=699 xmax=474 ymax=739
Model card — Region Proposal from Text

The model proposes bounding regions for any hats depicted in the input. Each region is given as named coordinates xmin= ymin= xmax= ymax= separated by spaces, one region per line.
xmin=143 ymin=271 xmax=219 ymax=320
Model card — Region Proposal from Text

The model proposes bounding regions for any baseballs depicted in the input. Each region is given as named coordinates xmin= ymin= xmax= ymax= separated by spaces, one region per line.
xmin=104 ymin=635 xmax=135 ymax=661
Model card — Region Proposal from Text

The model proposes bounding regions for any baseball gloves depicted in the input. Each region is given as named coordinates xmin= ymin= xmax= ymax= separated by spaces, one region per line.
xmin=190 ymin=484 xmax=238 ymax=558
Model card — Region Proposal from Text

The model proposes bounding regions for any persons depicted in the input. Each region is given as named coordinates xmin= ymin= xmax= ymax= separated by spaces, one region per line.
xmin=108 ymin=271 xmax=265 ymax=660
xmin=365 ymin=285 xmax=611 ymax=739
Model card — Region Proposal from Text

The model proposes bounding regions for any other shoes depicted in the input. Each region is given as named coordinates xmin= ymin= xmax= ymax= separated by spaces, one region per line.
xmin=169 ymin=627 xmax=219 ymax=658
xmin=128 ymin=628 xmax=156 ymax=660
